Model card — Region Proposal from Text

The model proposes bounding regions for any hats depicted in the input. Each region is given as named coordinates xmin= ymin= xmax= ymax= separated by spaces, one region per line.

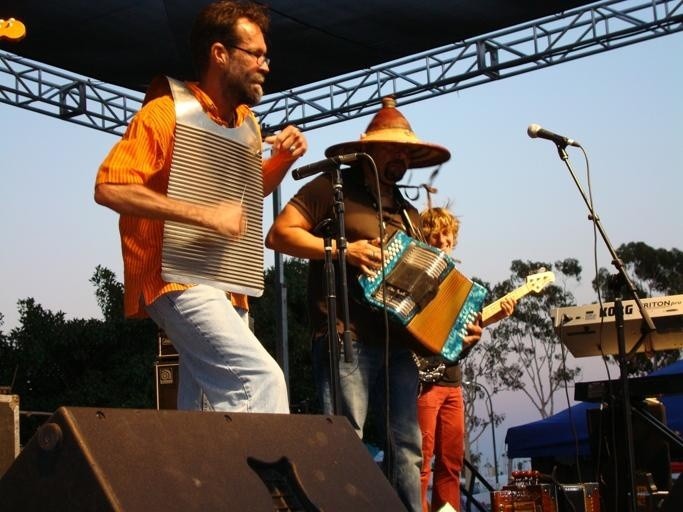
xmin=324 ymin=98 xmax=452 ymax=170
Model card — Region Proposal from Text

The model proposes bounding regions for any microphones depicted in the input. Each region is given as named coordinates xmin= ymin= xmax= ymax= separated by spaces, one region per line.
xmin=291 ymin=152 xmax=365 ymax=180
xmin=527 ymin=123 xmax=580 ymax=147
xmin=423 ymin=182 xmax=437 ymax=194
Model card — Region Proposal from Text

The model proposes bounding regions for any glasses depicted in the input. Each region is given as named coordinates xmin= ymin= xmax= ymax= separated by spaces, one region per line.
xmin=235 ymin=46 xmax=271 ymax=67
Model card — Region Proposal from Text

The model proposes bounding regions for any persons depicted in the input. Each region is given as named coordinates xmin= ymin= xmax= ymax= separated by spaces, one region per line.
xmin=264 ymin=97 xmax=452 ymax=512
xmin=95 ymin=0 xmax=307 ymax=415
xmin=417 ymin=208 xmax=517 ymax=512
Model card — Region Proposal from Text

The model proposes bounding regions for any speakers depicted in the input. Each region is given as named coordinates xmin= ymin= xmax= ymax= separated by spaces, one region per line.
xmin=0 ymin=406 xmax=409 ymax=512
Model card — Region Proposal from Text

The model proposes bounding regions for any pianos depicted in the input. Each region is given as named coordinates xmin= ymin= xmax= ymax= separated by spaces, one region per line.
xmin=548 ymin=294 xmax=682 ymax=357
xmin=574 ymin=372 xmax=682 ymax=404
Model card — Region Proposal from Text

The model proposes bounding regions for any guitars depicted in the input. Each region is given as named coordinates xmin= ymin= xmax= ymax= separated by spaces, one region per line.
xmin=412 ymin=267 xmax=556 ymax=385
